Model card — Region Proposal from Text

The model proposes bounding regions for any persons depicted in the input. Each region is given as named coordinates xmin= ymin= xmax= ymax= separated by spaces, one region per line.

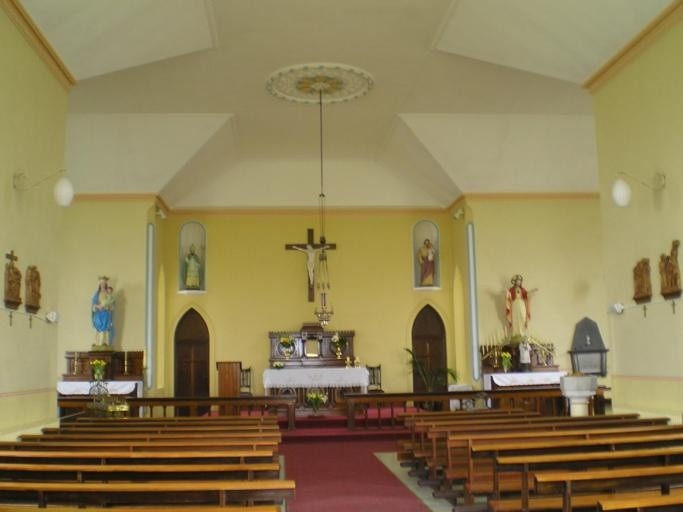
xmin=426 ymin=243 xmax=434 ymax=261
xmin=94 ymin=286 xmax=114 ymax=311
xmin=90 ymin=276 xmax=117 ymax=352
xmin=518 ymin=338 xmax=531 ymax=372
xmin=183 ymin=243 xmax=204 ymax=290
xmin=503 ymin=273 xmax=540 ymax=342
xmin=289 ymin=244 xmax=331 ymax=288
xmin=417 ymin=239 xmax=438 ymax=288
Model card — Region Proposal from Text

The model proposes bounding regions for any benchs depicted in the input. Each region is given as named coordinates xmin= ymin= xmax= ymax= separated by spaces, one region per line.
xmin=0 ymin=410 xmax=295 ymax=512
xmin=394 ymin=410 xmax=682 ymax=512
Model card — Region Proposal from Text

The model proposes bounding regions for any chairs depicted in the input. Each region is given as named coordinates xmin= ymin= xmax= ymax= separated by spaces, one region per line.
xmin=85 ymin=380 xmax=109 ymax=412
xmin=240 ymin=366 xmax=252 ymax=416
xmin=364 ymin=363 xmax=384 ymax=393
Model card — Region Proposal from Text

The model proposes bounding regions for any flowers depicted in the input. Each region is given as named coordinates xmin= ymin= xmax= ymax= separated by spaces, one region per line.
xmin=329 ymin=331 xmax=347 ymax=347
xmin=500 ymin=351 xmax=511 ymax=373
xmin=279 ymin=334 xmax=295 ymax=349
xmin=89 ymin=357 xmax=107 ymax=381
xmin=271 ymin=360 xmax=283 ymax=368
xmin=305 ymin=391 xmax=327 ymax=413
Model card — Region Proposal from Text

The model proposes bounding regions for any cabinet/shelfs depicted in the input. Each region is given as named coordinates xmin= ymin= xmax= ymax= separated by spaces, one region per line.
xmin=56 ymin=378 xmax=144 ymax=422
xmin=262 ymin=368 xmax=368 ymax=411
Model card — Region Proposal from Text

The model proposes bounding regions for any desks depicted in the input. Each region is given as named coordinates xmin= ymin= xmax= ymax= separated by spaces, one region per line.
xmin=446 ymin=384 xmax=473 ymax=413
xmin=481 ymin=371 xmax=568 ymax=416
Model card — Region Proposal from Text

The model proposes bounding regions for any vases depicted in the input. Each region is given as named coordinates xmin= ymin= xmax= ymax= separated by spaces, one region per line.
xmin=283 ymin=347 xmax=291 ymax=359
xmin=312 ymin=407 xmax=319 ymax=416
xmin=334 ymin=346 xmax=342 ymax=360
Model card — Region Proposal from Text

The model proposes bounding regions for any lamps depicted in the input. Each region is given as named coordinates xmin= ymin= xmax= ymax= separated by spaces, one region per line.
xmin=11 ymin=165 xmax=76 ymax=209
xmin=453 ymin=208 xmax=464 ymax=220
xmin=611 ymin=169 xmax=665 ymax=209
xmin=265 ymin=57 xmax=374 ymax=330
xmin=155 ymin=207 xmax=167 ymax=220
xmin=610 ymin=300 xmax=643 ymax=315
xmin=35 ymin=311 xmax=60 ymax=324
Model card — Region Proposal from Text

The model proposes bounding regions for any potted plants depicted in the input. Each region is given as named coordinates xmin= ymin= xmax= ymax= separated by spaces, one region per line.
xmin=402 ymin=347 xmax=456 ymax=410
